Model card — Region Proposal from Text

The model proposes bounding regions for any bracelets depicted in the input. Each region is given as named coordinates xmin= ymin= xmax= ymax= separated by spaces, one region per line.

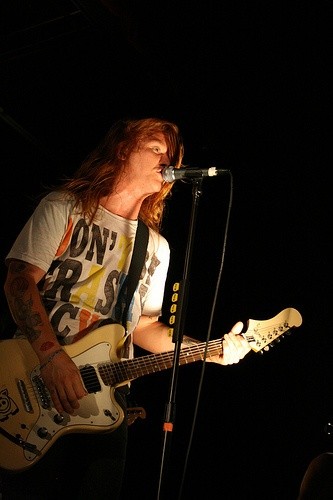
xmin=37 ymin=348 xmax=62 ymax=372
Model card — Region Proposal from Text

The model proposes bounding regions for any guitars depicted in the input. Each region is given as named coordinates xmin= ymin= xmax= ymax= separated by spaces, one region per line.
xmin=0 ymin=307 xmax=303 ymax=469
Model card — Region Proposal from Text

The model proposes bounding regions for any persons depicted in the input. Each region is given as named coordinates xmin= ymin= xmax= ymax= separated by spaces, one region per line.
xmin=2 ymin=115 xmax=250 ymax=499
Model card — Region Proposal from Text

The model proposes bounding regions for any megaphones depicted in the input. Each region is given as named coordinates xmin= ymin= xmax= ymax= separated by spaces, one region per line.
xmin=162 ymin=165 xmax=230 ymax=183
